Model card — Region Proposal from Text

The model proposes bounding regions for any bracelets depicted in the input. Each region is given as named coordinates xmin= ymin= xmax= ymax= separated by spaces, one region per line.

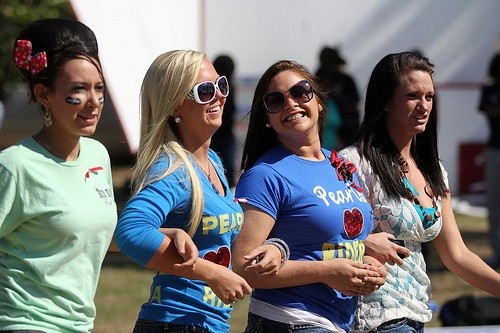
xmin=271 ymin=242 xmax=285 ymax=268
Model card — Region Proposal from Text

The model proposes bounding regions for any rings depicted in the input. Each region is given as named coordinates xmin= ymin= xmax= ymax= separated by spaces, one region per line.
xmin=231 ymin=300 xmax=236 ymax=303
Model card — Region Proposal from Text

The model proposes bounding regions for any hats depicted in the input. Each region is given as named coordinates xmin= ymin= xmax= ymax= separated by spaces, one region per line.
xmin=319 ymin=46 xmax=346 ymax=63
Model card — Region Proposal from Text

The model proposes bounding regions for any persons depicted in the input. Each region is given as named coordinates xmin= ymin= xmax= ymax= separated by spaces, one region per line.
xmin=313 ymin=48 xmax=359 ymax=152
xmin=232 ymin=60 xmax=386 ymax=333
xmin=210 ymin=54 xmax=234 ymax=163
xmin=0 ymin=19 xmax=199 ymax=333
xmin=115 ymin=49 xmax=252 ymax=333
xmin=337 ymin=52 xmax=500 ymax=333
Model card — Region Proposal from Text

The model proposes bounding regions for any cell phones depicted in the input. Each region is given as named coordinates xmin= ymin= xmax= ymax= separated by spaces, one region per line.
xmin=389 ymin=239 xmax=406 ymax=259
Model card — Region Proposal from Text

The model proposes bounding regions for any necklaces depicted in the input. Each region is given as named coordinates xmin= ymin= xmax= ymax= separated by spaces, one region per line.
xmin=42 ymin=142 xmax=80 ymax=161
xmin=196 ymin=161 xmax=220 ymax=194
xmin=392 ymin=152 xmax=441 ymax=220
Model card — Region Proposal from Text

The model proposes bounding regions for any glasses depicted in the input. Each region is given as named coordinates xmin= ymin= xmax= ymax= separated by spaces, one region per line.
xmin=262 ymin=79 xmax=313 ymax=114
xmin=182 ymin=75 xmax=230 ymax=105
